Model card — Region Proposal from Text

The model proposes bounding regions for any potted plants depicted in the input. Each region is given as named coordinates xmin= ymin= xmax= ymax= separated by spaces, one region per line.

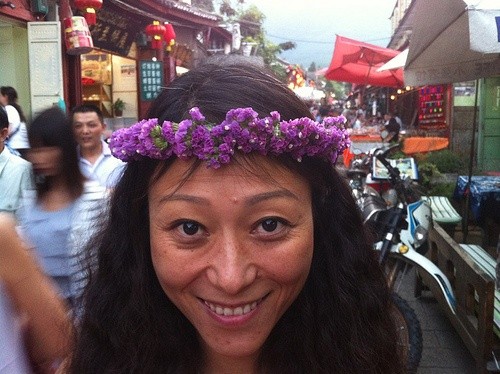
xmin=112 ymin=98 xmax=127 ymax=117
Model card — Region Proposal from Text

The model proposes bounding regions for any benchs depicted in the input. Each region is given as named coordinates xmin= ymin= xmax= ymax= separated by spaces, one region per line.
xmin=421 ymin=195 xmax=500 ymax=374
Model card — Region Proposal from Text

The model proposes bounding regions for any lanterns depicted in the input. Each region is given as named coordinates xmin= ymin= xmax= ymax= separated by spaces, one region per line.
xmin=76 ymin=0 xmax=103 ymax=24
xmin=146 ymin=20 xmax=175 ymax=51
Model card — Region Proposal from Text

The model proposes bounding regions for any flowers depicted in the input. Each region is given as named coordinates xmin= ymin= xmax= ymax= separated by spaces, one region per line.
xmin=104 ymin=107 xmax=353 ymax=170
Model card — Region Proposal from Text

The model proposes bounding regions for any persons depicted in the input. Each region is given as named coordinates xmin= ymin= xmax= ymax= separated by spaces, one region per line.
xmin=0 ymin=86 xmax=127 ymax=374
xmin=316 ymin=98 xmax=402 ymax=143
xmin=70 ymin=59 xmax=405 ymax=374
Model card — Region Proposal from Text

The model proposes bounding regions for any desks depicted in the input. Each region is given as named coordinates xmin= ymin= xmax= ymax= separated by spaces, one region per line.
xmin=456 ymin=174 xmax=500 ymax=250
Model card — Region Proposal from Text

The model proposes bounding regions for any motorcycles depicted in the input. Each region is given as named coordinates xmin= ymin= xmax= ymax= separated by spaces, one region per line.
xmin=344 ymin=116 xmax=425 ymax=372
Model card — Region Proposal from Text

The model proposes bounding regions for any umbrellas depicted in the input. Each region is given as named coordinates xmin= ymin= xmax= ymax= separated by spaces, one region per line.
xmin=323 ymin=34 xmax=405 ymax=103
